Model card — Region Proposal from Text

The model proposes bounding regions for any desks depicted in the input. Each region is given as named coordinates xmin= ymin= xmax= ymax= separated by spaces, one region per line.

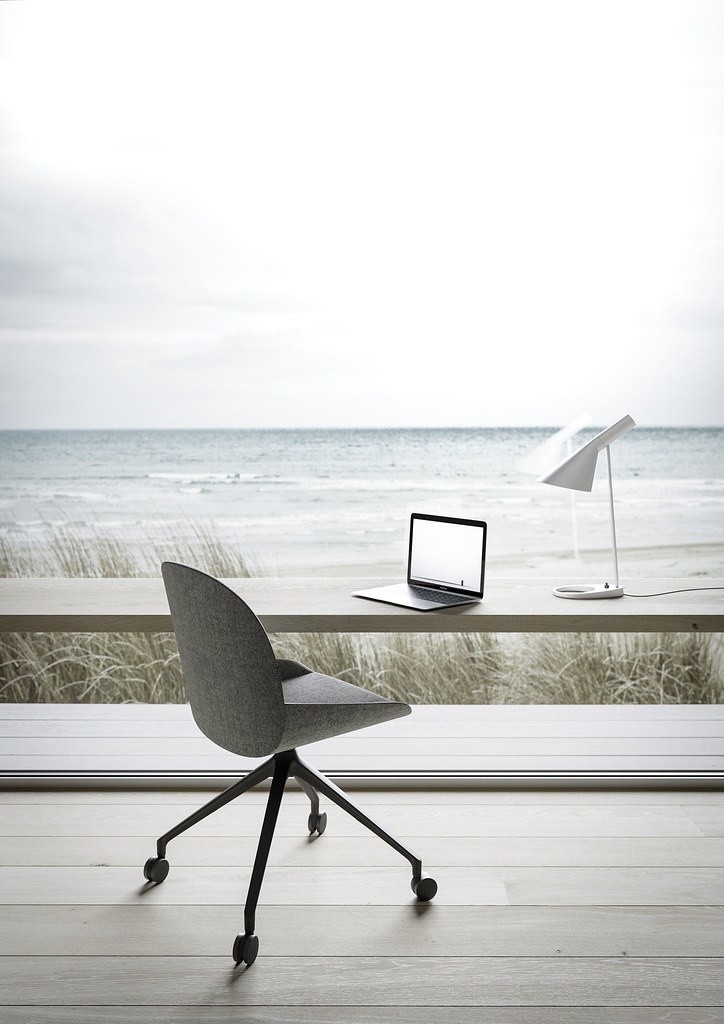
xmin=0 ymin=577 xmax=724 ymax=632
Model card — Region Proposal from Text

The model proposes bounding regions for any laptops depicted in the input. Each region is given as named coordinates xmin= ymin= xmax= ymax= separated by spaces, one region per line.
xmin=351 ymin=512 xmax=487 ymax=612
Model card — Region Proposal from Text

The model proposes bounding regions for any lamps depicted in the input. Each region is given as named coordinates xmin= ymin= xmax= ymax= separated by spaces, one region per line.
xmin=536 ymin=414 xmax=636 ymax=601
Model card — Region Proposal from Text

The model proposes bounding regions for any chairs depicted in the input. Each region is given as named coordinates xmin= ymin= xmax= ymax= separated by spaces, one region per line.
xmin=142 ymin=559 xmax=437 ymax=965
